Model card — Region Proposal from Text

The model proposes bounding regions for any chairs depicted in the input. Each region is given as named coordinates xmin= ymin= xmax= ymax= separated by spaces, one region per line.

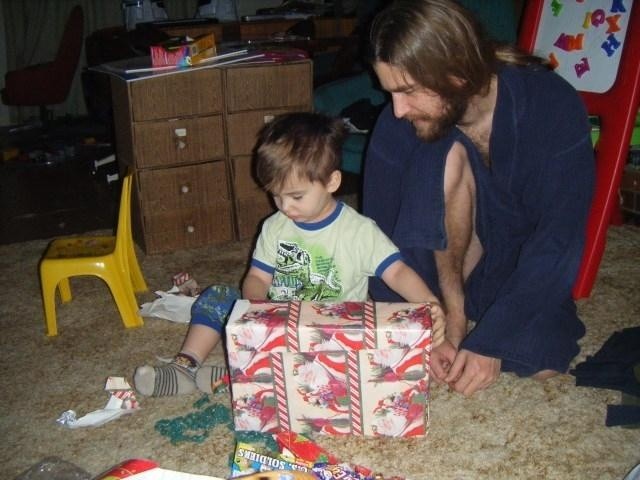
xmin=2 ymin=5 xmax=86 ymax=134
xmin=38 ymin=174 xmax=145 ymax=337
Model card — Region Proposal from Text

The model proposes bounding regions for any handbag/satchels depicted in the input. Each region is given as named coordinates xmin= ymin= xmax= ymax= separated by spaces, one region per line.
xmin=569 ymin=326 xmax=640 ymax=396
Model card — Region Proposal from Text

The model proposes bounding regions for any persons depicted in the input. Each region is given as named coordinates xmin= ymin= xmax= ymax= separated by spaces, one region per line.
xmin=358 ymin=1 xmax=599 ymax=397
xmin=133 ymin=108 xmax=445 ymax=397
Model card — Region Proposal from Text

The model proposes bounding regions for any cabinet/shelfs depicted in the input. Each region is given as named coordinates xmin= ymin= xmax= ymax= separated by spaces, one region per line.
xmin=224 ymin=61 xmax=315 ymax=244
xmin=235 ymin=15 xmax=363 ymax=53
xmin=104 ymin=69 xmax=234 ymax=252
xmin=152 ymin=23 xmax=226 ymax=41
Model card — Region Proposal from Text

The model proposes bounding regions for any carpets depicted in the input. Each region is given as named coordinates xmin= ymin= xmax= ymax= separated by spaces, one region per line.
xmin=1 ymin=112 xmax=121 ymax=243
xmin=0 ymin=224 xmax=640 ymax=479
